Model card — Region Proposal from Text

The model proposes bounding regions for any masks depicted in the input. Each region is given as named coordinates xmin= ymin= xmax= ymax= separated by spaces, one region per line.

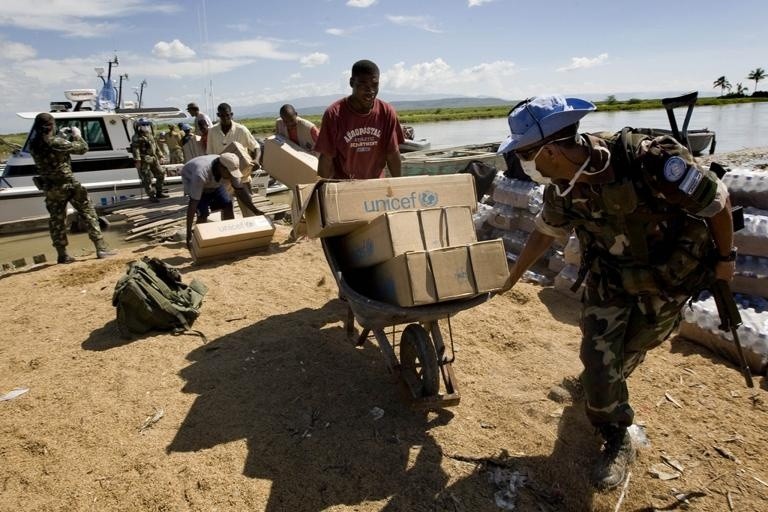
xmin=519 ymin=142 xmax=553 ymax=185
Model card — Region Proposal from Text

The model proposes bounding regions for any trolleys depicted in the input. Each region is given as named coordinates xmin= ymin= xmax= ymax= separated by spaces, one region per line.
xmin=306 ymin=210 xmax=493 ymax=411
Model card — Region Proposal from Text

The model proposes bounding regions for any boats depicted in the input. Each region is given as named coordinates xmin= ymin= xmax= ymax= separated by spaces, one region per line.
xmin=397 ymin=134 xmax=434 ymax=153
xmin=1 ymin=50 xmax=190 ymax=221
xmin=641 ymin=122 xmax=717 ymax=155
xmin=383 ymin=139 xmax=507 ymax=189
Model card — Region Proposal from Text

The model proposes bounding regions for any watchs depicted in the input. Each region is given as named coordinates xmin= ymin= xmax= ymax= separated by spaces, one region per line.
xmin=720 ymin=247 xmax=738 ymax=261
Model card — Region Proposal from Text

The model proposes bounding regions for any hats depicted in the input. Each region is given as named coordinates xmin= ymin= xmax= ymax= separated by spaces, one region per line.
xmin=496 ymin=96 xmax=597 ymax=155
xmin=220 ymin=152 xmax=243 ymax=178
xmin=136 ymin=119 xmax=151 ymax=125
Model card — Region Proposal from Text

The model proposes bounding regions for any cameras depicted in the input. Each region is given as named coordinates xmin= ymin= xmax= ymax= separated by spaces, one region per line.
xmin=65 ymin=129 xmax=72 ymax=134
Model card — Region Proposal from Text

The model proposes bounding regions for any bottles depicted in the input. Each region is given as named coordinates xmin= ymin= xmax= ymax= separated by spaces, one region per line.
xmin=470 ymin=171 xmax=587 ymax=301
xmin=686 ymin=161 xmax=768 ymax=370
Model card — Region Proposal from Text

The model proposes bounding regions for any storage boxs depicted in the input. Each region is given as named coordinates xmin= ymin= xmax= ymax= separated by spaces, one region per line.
xmin=190 ymin=214 xmax=274 ymax=263
xmin=341 ymin=204 xmax=477 ymax=269
xmin=261 ymin=135 xmax=318 ymax=188
xmin=292 ymin=169 xmax=479 ymax=237
xmin=371 ymin=237 xmax=509 ymax=308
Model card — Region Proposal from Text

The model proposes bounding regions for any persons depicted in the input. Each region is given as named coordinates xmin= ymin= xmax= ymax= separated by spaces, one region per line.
xmin=29 ymin=113 xmax=119 ymax=264
xmin=276 ymin=104 xmax=321 ymax=157
xmin=312 ymin=60 xmax=405 ymax=179
xmin=496 ymin=96 xmax=734 ymax=490
xmin=131 ymin=102 xmax=264 ymax=254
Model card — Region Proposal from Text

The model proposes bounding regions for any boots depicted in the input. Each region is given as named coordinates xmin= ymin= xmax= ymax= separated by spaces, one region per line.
xmin=549 ymin=375 xmax=584 ymax=402
xmin=590 ymin=426 xmax=635 ymax=489
xmin=96 ymin=238 xmax=118 ymax=258
xmin=150 ymin=191 xmax=169 ymax=203
xmin=57 ymin=247 xmax=75 ymax=262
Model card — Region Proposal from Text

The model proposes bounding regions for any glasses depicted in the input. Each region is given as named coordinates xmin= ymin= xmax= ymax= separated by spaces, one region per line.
xmin=519 ymin=143 xmax=550 ymax=160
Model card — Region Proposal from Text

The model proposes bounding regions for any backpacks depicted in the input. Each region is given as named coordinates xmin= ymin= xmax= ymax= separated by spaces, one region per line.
xmin=112 ymin=257 xmax=208 ymax=341
